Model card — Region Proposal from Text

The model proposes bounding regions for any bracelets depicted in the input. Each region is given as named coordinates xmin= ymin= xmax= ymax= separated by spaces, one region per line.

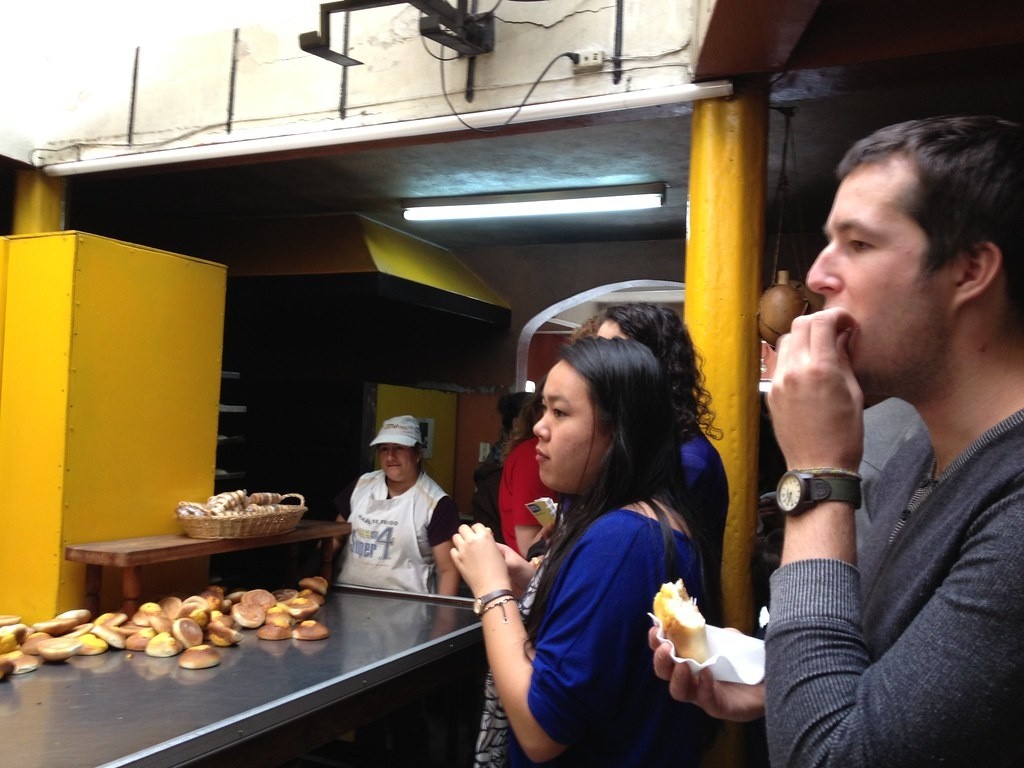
xmin=786 ymin=466 xmax=863 ymax=481
xmin=481 ymin=598 xmax=519 ymax=624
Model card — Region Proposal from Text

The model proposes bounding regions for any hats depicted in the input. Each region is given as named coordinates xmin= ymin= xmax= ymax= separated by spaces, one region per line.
xmin=369 ymin=415 xmax=425 ymax=446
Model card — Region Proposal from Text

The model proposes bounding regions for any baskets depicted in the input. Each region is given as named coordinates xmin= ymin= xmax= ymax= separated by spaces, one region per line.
xmin=175 ymin=493 xmax=307 ymax=539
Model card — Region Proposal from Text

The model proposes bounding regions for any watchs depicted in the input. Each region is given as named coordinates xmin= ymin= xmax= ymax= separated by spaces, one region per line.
xmin=473 ymin=589 xmax=519 ymax=616
xmin=774 ymin=470 xmax=862 ymax=515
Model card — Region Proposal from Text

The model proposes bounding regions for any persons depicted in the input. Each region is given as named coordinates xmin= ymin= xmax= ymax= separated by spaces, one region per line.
xmin=449 ymin=301 xmax=729 ymax=768
xmin=319 ymin=412 xmax=462 ymax=598
xmin=646 ymin=115 xmax=1024 ymax=767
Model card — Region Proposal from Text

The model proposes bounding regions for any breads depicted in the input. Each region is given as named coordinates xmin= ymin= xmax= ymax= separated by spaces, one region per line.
xmin=0 ymin=576 xmax=326 ymax=682
xmin=653 ymin=578 xmax=712 ymax=663
xmin=180 ymin=489 xmax=286 ymax=515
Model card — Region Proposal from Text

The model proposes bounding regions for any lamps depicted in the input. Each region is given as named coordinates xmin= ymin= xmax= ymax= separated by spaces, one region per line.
xmin=401 ymin=182 xmax=673 ymax=221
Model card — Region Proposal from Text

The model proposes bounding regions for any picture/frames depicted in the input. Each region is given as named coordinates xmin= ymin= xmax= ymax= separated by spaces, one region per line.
xmin=418 ymin=417 xmax=435 ymax=460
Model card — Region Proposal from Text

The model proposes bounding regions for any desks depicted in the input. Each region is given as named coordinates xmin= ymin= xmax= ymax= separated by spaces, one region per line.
xmin=61 ymin=519 xmax=351 ymax=627
